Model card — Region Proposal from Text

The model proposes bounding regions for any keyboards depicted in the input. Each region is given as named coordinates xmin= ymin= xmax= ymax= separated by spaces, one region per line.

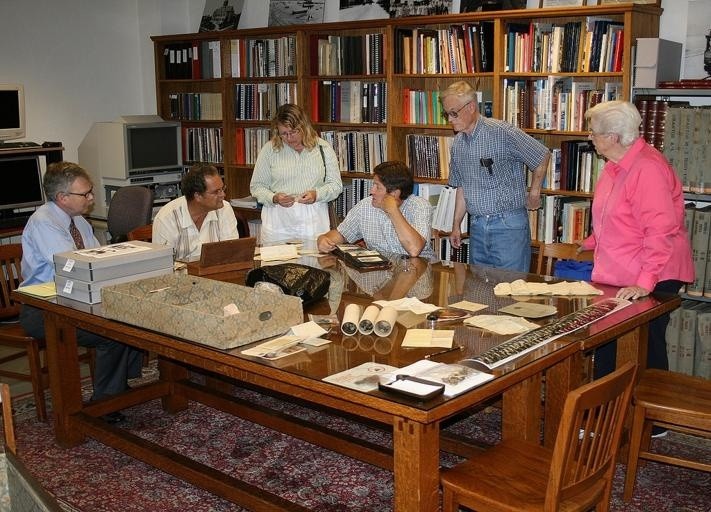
xmin=0 ymin=216 xmax=30 ymax=227
xmin=0 ymin=142 xmax=42 ymax=150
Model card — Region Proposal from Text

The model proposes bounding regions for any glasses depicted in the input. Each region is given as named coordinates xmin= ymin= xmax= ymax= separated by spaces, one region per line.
xmin=440 ymin=101 xmax=472 ymax=122
xmin=66 ymin=185 xmax=94 ymax=199
xmin=201 ymin=184 xmax=227 ymax=195
xmin=280 ymin=129 xmax=301 ymax=138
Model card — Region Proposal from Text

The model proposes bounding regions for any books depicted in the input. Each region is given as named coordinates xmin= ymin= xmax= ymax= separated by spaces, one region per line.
xmin=498 ymin=18 xmax=624 ymax=245
xmin=162 ymin=40 xmax=224 ymax=188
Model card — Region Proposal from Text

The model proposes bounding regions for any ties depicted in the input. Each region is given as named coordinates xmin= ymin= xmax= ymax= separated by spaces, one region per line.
xmin=68 ymin=216 xmax=85 ymax=249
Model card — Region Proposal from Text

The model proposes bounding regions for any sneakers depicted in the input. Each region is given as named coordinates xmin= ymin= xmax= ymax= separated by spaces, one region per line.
xmin=649 ymin=424 xmax=670 ymax=439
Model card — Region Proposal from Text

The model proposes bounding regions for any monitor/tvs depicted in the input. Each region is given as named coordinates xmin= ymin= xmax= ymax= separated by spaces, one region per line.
xmin=122 ymin=121 xmax=184 ymax=179
xmin=0 ymin=82 xmax=26 ymax=142
xmin=0 ymin=155 xmax=45 ymax=218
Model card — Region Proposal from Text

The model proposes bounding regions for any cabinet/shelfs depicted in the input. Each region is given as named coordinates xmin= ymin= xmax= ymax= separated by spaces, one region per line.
xmin=391 ymin=11 xmax=501 ymax=241
xmin=154 ymin=36 xmax=228 ymax=199
xmin=228 ymin=27 xmax=305 ymax=225
xmin=305 ymin=20 xmax=391 ymax=225
xmin=500 ymin=6 xmax=665 ymax=244
xmin=628 ymin=87 xmax=711 ymax=306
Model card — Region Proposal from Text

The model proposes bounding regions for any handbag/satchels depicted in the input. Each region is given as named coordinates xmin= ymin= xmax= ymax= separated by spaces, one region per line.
xmin=245 ymin=262 xmax=332 ymax=307
xmin=328 ymin=201 xmax=338 ymax=231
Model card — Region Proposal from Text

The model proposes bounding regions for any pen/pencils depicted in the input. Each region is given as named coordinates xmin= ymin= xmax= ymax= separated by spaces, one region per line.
xmin=424 ymin=346 xmax=460 ymax=359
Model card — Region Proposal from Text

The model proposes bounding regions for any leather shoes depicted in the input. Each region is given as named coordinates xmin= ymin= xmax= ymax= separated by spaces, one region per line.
xmin=95 ymin=412 xmax=126 ymax=423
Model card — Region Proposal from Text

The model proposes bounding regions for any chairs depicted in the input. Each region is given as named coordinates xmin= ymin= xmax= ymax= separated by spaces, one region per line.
xmin=539 ymin=243 xmax=596 ymax=280
xmin=0 ymin=242 xmax=99 ymax=424
xmin=441 ymin=359 xmax=640 ymax=512
xmin=106 ymin=184 xmax=154 ymax=248
xmin=126 ymin=224 xmax=154 ymax=370
xmin=624 ymin=365 xmax=711 ymax=502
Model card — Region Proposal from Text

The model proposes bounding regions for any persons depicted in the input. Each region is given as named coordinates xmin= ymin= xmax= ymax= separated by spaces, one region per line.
xmin=438 ymin=82 xmax=551 ymax=273
xmin=334 ymin=259 xmax=535 ymax=356
xmin=573 ymin=100 xmax=695 ymax=444
xmin=17 ymin=160 xmax=140 ymax=424
xmin=152 ymin=163 xmax=240 ymax=265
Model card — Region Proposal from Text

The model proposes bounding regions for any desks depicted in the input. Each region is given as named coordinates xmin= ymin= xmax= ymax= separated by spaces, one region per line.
xmin=10 ymin=238 xmax=684 ymax=512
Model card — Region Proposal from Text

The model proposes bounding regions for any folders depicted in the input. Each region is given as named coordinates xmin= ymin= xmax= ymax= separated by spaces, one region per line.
xmin=164 ymin=42 xmax=201 ymax=80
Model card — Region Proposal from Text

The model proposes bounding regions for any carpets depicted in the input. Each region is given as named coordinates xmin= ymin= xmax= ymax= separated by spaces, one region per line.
xmin=0 ymin=356 xmax=711 ymax=511
